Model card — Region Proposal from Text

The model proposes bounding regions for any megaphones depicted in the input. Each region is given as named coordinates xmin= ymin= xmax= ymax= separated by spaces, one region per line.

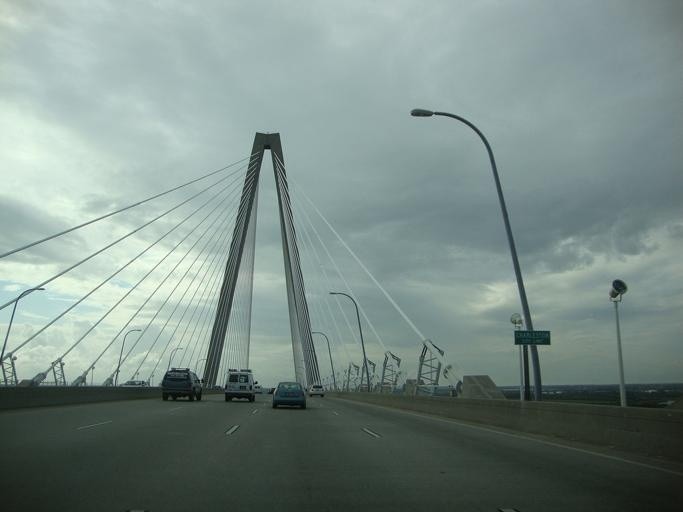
xmin=511 ymin=313 xmax=522 ymax=326
xmin=609 ymin=278 xmax=628 ymax=298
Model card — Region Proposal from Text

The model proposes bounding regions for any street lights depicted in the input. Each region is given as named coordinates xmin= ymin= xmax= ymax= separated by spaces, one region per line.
xmin=298 ymin=331 xmax=336 ymax=389
xmin=115 ymin=329 xmax=142 ymax=387
xmin=409 ymin=109 xmax=542 ymax=400
xmin=0 ymin=287 xmax=45 ymax=362
xmin=168 ymin=348 xmax=183 ymax=369
xmin=330 ymin=292 xmax=370 ymax=392
xmin=511 ymin=313 xmax=524 ymax=401
xmin=609 ymin=280 xmax=627 ymax=407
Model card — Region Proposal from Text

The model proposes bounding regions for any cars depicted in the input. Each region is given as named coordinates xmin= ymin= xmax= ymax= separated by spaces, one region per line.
xmin=162 ymin=368 xmax=204 ymax=401
xmin=309 ymin=384 xmax=324 ymax=397
xmin=121 ymin=381 xmax=149 ymax=387
xmin=271 ymin=382 xmax=307 ymax=408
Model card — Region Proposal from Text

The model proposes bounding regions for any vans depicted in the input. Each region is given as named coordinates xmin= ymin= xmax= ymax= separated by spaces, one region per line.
xmin=224 ymin=369 xmax=262 ymax=402
